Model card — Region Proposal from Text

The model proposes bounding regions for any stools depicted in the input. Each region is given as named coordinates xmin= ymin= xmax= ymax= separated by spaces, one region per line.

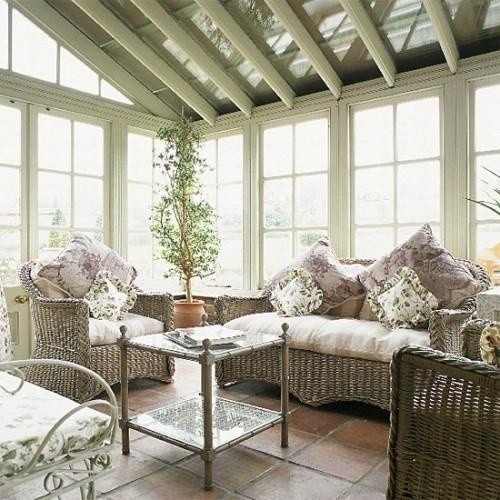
xmin=0 ymin=359 xmax=118 ymax=500
xmin=115 ymin=323 xmax=292 ymax=490
xmin=384 ymin=316 xmax=500 ymax=500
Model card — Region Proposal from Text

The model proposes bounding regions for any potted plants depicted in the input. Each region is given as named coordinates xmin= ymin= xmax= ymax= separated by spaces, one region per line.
xmin=150 ymin=104 xmax=220 ymax=330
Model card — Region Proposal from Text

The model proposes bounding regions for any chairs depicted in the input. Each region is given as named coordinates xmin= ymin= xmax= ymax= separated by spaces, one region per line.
xmin=17 ymin=258 xmax=176 ymax=403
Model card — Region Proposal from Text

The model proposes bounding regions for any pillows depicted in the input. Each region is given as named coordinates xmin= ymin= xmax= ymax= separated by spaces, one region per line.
xmin=261 ymin=221 xmax=487 ymax=332
xmin=28 ymin=230 xmax=144 ymax=321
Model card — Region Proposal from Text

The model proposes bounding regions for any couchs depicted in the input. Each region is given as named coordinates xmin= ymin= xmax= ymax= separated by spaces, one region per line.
xmin=212 ymin=256 xmax=494 ymax=414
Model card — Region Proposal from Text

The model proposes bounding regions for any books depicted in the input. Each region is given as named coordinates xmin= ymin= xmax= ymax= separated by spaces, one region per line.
xmin=162 ymin=324 xmax=248 ymax=348
